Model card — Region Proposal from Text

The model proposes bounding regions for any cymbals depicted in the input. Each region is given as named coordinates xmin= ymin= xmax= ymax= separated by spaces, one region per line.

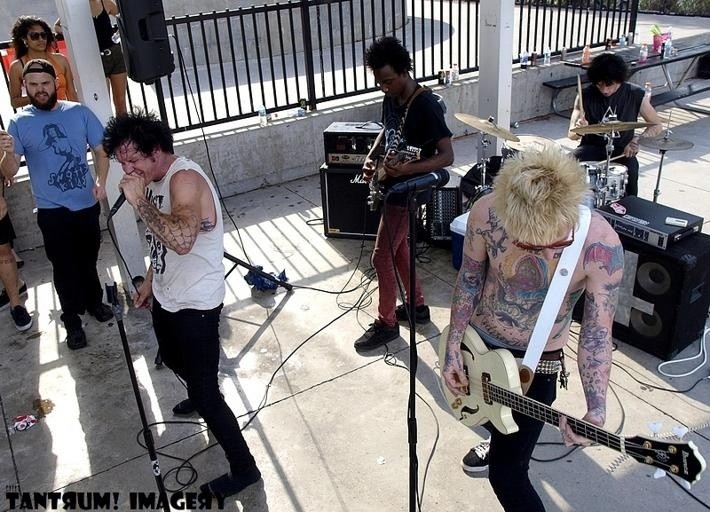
xmin=453 ymin=112 xmax=521 ymax=145
xmin=635 ymin=134 xmax=695 ymax=152
xmin=568 ymin=120 xmax=659 ymax=135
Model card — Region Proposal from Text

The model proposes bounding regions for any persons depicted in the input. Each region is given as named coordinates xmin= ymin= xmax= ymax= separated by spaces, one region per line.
xmin=567 ymin=53 xmax=662 ymax=199
xmin=441 ymin=145 xmax=627 ymax=511
xmin=352 ymin=36 xmax=456 ymax=352
xmin=102 ymin=109 xmax=261 ymax=500
xmin=0 ymin=0 xmax=130 ymax=352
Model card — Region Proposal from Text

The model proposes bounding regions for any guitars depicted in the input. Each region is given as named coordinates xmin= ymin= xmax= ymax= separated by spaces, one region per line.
xmin=366 ymin=143 xmax=424 ymax=212
xmin=436 ymin=323 xmax=708 ymax=490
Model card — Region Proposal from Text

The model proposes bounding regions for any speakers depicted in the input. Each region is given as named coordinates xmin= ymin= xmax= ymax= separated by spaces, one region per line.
xmin=319 ymin=163 xmax=378 ymax=239
xmin=116 ymin=0 xmax=175 ymax=83
xmin=617 ymin=236 xmax=709 ymax=359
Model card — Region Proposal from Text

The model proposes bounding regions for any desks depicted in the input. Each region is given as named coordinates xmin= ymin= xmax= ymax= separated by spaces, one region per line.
xmin=564 ymin=42 xmax=710 ymax=116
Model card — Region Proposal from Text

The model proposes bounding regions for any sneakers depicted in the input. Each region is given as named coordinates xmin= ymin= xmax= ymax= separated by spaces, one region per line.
xmin=355 ymin=319 xmax=400 ymax=351
xmin=395 ymin=304 xmax=430 ymax=324
xmin=172 ymin=399 xmax=196 ymax=415
xmin=461 ymin=436 xmax=492 ymax=472
xmin=10 ymin=305 xmax=32 ymax=331
xmin=88 ymin=302 xmax=113 ymax=322
xmin=64 ymin=316 xmax=87 ymax=349
xmin=0 ymin=281 xmax=27 ymax=311
xmin=200 ymin=473 xmax=242 ymax=499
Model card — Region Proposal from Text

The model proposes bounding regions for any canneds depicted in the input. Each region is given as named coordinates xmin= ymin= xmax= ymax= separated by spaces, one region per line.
xmin=530 ymin=52 xmax=537 ymax=66
xmin=438 ymin=71 xmax=445 ymax=85
xmin=628 ymin=32 xmax=634 ymax=46
xmin=561 ymin=47 xmax=567 ymax=61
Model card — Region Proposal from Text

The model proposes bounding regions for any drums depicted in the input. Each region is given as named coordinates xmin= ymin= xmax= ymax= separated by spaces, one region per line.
xmin=575 ymin=159 xmax=631 ymax=209
xmin=499 ymin=132 xmax=562 ymax=162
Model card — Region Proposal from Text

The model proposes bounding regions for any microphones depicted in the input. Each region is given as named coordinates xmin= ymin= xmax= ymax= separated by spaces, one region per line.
xmin=392 ymin=169 xmax=451 ymax=196
xmin=109 ymin=188 xmax=128 ymax=215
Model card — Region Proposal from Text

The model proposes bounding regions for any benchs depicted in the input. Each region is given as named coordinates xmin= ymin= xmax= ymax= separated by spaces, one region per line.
xmin=543 ymin=78 xmax=710 ymax=121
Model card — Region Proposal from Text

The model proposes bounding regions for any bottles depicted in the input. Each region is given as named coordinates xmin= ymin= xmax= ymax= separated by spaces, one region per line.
xmin=582 ymin=44 xmax=591 ymax=64
xmin=438 ymin=64 xmax=458 ymax=85
xmin=664 ymin=38 xmax=672 ymax=58
xmin=620 ymin=34 xmax=625 ymax=48
xmin=639 ymin=43 xmax=648 ymax=63
xmin=644 ymin=81 xmax=652 ymax=103
xmin=259 ymin=104 xmax=268 ymax=128
xmin=520 ymin=47 xmax=567 ymax=68
xmin=660 ymin=42 xmax=665 ymax=58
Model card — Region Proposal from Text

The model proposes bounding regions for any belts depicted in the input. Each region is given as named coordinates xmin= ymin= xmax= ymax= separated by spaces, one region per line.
xmin=535 ymin=360 xmax=561 ymax=375
xmin=100 ymin=44 xmax=121 ymax=56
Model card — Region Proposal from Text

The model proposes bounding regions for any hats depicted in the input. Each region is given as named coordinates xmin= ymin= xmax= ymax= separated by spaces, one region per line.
xmin=22 ymin=58 xmax=56 ymax=79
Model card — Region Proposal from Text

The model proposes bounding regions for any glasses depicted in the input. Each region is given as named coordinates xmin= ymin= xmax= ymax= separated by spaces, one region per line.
xmin=512 ymin=229 xmax=574 ymax=251
xmin=28 ymin=31 xmax=48 ymax=40
